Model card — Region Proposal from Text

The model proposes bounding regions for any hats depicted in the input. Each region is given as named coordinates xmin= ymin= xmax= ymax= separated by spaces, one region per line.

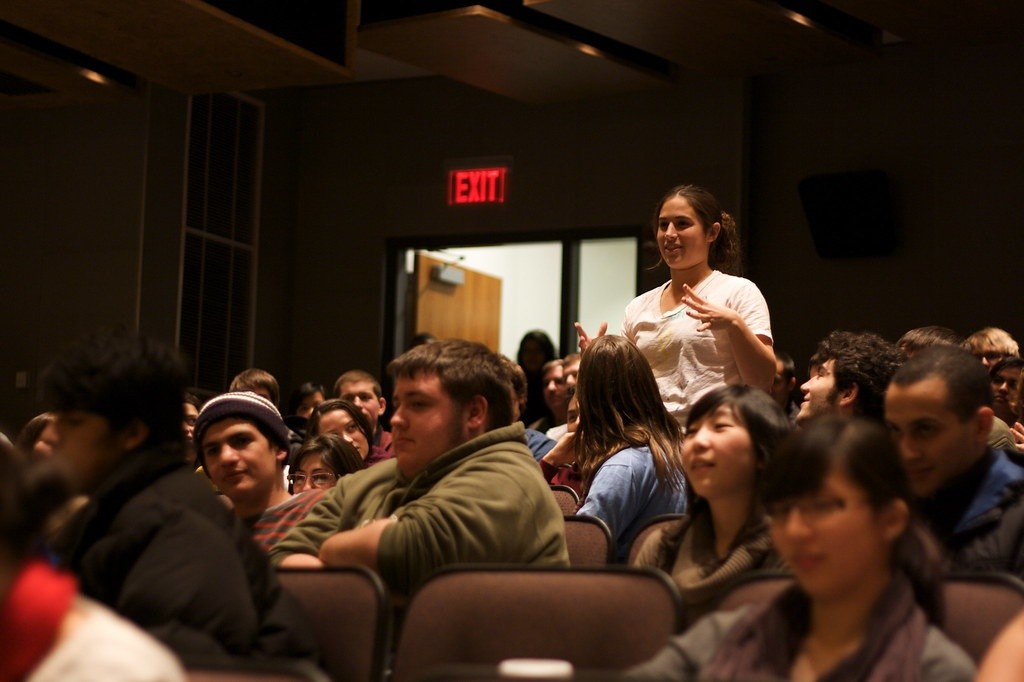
xmin=193 ymin=391 xmax=291 ymax=480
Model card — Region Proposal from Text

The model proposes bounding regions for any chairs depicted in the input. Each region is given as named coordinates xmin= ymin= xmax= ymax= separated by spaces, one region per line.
xmin=177 ymin=485 xmax=1023 ymax=681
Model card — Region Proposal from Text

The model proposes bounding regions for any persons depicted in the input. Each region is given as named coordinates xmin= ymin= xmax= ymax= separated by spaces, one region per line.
xmin=573 ymin=185 xmax=777 ymax=433
xmin=0 ymin=331 xmax=1024 ymax=682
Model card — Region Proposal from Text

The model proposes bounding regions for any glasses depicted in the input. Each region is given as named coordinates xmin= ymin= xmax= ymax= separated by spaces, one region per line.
xmin=287 ymin=473 xmax=340 ymax=485
